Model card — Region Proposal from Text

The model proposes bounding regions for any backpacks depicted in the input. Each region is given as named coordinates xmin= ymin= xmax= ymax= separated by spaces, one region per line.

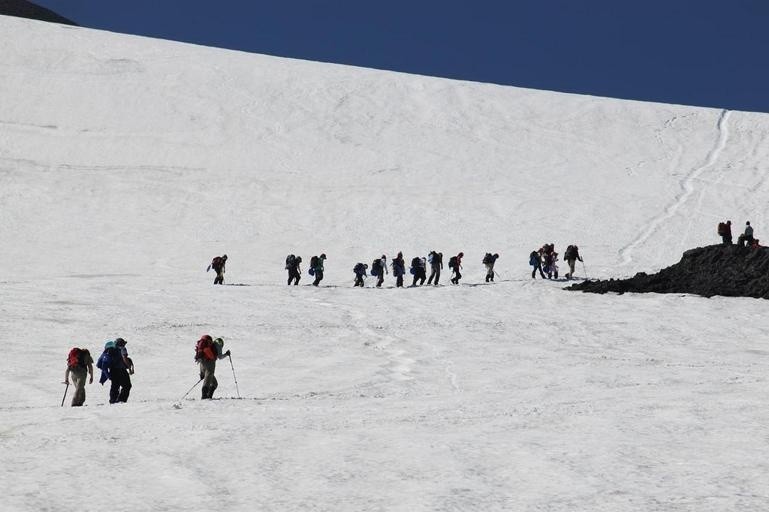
xmin=311 ymin=256 xmax=320 ymax=267
xmin=67 ymin=347 xmax=84 ymax=370
xmin=718 ymin=222 xmax=726 ymax=236
xmin=286 ymin=254 xmax=296 ymax=264
xmin=483 ymin=253 xmax=492 ymax=263
xmin=195 ymin=335 xmax=217 ymax=361
xmin=354 ymin=251 xmax=458 ymax=276
xmin=212 ymin=257 xmax=224 ymax=269
xmin=530 ymin=243 xmax=575 ymax=272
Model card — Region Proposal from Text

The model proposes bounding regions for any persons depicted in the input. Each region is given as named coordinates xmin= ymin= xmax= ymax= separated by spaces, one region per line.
xmin=531 ymin=242 xmax=583 ymax=280
xmin=64 ymin=348 xmax=93 ymax=406
xmin=108 ymin=338 xmax=134 ymax=402
xmin=486 ymin=253 xmax=498 ymax=281
xmin=428 ymin=252 xmax=443 ymax=285
xmin=723 ymin=220 xmax=732 ymax=242
xmin=452 ymin=252 xmax=464 ymax=283
xmin=312 ymin=254 xmax=326 ymax=286
xmin=213 ymin=254 xmax=228 ymax=285
xmin=199 ymin=338 xmax=229 ymax=398
xmin=412 ymin=257 xmax=426 ymax=286
xmin=738 ymin=221 xmax=753 ymax=246
xmin=354 ymin=264 xmax=368 ymax=286
xmin=288 ymin=257 xmax=302 ymax=285
xmin=377 ymin=255 xmax=388 ymax=287
xmin=397 ymin=251 xmax=406 ymax=286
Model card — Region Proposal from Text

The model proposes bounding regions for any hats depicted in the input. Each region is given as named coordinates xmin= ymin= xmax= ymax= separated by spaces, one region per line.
xmin=115 ymin=337 xmax=126 ymax=348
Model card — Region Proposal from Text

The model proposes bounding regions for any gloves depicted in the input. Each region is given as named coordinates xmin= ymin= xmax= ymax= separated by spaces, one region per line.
xmin=227 ymin=351 xmax=230 ymax=355
xmin=200 ymin=374 xmax=204 ymax=379
